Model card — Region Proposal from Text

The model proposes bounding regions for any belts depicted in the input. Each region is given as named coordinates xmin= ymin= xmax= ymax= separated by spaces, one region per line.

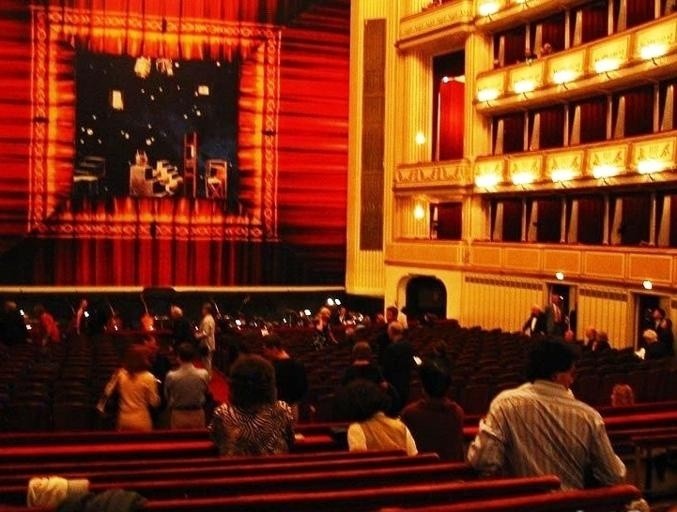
xmin=173 ymin=402 xmax=203 ymax=411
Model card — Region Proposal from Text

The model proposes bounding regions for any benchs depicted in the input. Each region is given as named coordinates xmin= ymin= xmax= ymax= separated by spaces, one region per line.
xmin=0 ymin=397 xmax=677 ymax=510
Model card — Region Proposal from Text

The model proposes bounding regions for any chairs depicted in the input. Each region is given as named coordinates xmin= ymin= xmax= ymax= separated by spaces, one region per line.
xmin=1 ymin=314 xmax=676 ymax=429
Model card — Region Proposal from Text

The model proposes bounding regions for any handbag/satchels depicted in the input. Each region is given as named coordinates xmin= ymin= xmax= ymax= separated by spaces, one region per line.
xmin=96 ymin=369 xmax=119 ymax=416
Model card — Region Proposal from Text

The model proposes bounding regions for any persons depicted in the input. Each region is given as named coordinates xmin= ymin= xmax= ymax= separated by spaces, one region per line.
xmin=33 ymin=305 xmax=61 ymax=346
xmin=522 ymin=294 xmax=674 ymax=360
xmin=119 ymin=303 xmax=465 ymax=458
xmin=468 ymin=340 xmax=627 ymax=492
xmin=66 ymin=299 xmax=88 ymax=340
